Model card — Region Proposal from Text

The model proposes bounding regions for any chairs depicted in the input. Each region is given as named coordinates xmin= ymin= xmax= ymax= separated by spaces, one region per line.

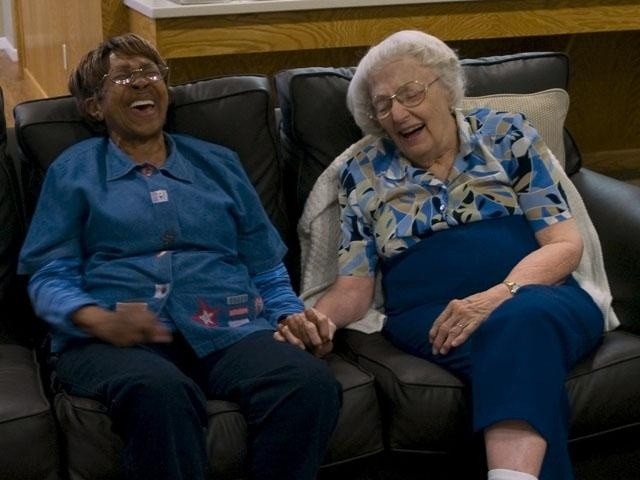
xmin=14 ymin=77 xmax=383 ymax=480
xmin=0 ymin=86 xmax=60 ymax=480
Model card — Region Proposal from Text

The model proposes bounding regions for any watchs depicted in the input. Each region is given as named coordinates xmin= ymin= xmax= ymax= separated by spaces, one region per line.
xmin=503 ymin=281 xmax=522 ymax=295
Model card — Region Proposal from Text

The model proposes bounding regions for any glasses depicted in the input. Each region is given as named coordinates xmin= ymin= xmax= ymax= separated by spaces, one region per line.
xmin=96 ymin=59 xmax=175 ymax=97
xmin=363 ymin=72 xmax=447 ymax=125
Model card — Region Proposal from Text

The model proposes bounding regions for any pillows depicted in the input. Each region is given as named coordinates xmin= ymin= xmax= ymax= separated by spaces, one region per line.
xmin=457 ymin=86 xmax=569 ymax=173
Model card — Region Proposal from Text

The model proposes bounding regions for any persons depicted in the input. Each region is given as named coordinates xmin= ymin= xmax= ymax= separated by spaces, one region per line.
xmin=18 ymin=34 xmax=342 ymax=480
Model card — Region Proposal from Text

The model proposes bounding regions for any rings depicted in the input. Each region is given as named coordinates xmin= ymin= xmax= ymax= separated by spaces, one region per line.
xmin=457 ymin=322 xmax=465 ymax=330
